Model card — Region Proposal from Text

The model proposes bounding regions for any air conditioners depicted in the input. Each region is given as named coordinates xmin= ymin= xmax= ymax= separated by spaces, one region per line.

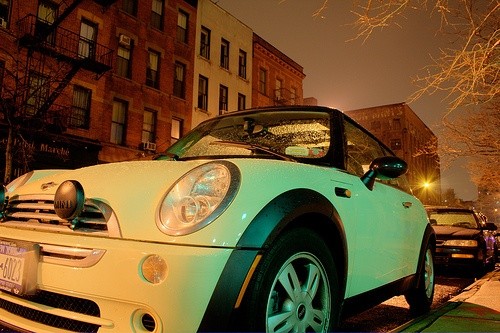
xmin=119 ymin=35 xmax=130 ymax=47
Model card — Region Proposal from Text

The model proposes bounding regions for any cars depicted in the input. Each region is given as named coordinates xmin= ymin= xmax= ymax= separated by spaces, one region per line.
xmin=421 ymin=203 xmax=498 ymax=276
xmin=0 ymin=105 xmax=438 ymax=333
